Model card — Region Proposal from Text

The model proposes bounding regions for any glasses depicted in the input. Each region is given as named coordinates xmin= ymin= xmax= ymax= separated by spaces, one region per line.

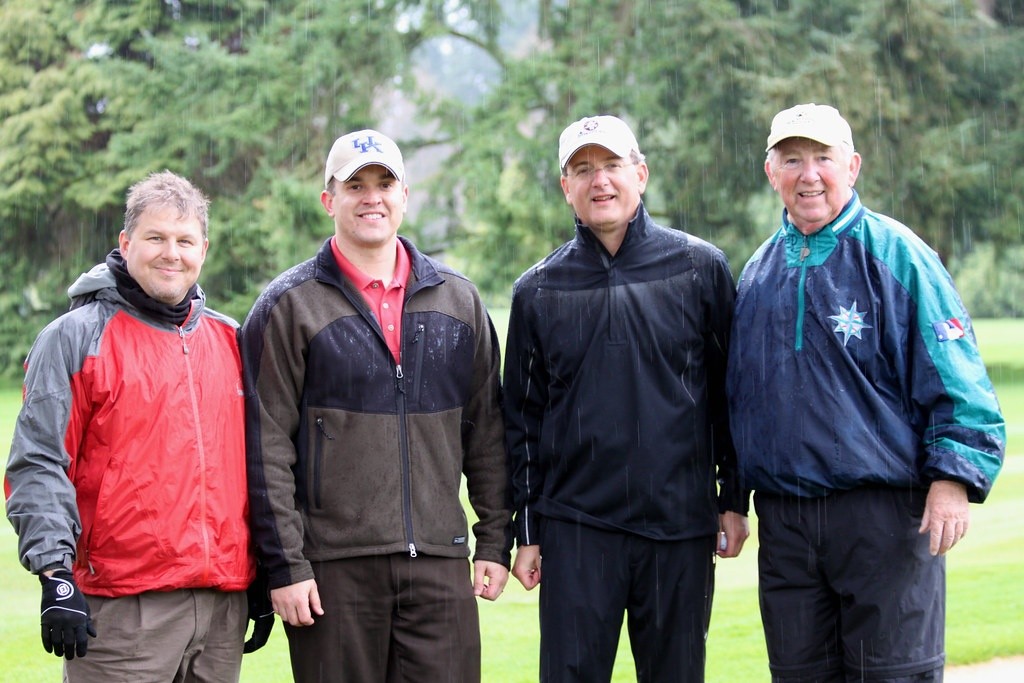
xmin=566 ymin=157 xmax=638 ymax=181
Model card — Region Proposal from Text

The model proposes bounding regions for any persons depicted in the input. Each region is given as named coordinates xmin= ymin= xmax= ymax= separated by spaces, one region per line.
xmin=724 ymin=102 xmax=1005 ymax=683
xmin=502 ymin=110 xmax=753 ymax=682
xmin=238 ymin=128 xmax=517 ymax=682
xmin=2 ymin=167 xmax=274 ymax=682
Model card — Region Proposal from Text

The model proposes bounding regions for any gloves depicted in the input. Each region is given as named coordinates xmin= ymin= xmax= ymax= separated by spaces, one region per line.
xmin=240 ymin=579 xmax=273 ymax=651
xmin=36 ymin=568 xmax=97 ymax=657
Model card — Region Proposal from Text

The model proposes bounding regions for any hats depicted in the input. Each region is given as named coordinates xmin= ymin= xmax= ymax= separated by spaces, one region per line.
xmin=765 ymin=98 xmax=852 ymax=162
xmin=322 ymin=131 xmax=403 ymax=190
xmin=554 ymin=116 xmax=643 ymax=173
